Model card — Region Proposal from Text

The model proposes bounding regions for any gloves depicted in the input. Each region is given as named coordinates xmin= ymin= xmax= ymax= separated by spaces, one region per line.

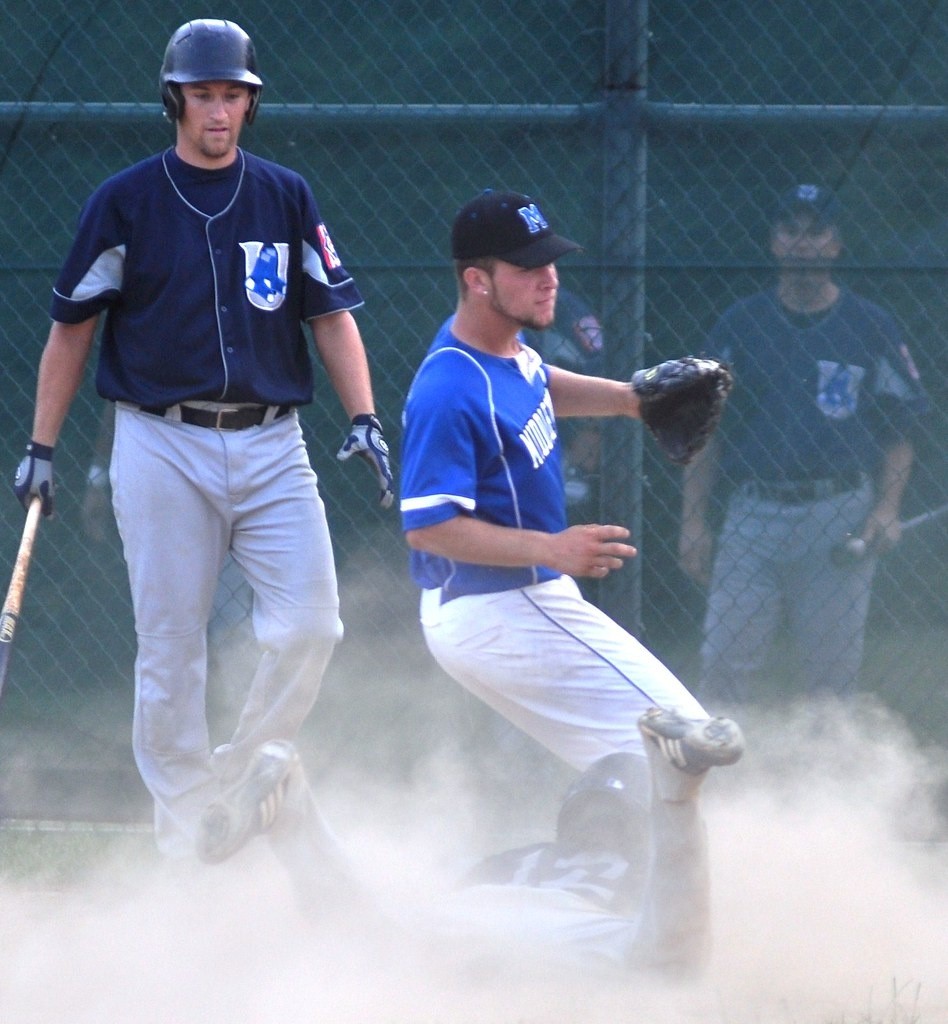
xmin=337 ymin=415 xmax=395 ymax=509
xmin=12 ymin=441 xmax=55 ymax=521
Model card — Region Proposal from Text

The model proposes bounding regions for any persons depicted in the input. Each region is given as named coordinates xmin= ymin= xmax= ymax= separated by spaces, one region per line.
xmin=519 ymin=275 xmax=606 ymax=605
xmin=192 ymin=703 xmax=749 ymax=993
xmin=677 ymin=181 xmax=934 ymax=704
xmin=401 ymin=186 xmax=733 ymax=794
xmin=10 ymin=19 xmax=393 ymax=893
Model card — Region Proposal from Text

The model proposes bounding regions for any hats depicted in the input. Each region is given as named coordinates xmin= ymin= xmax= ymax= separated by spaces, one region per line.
xmin=771 ymin=180 xmax=845 ymax=227
xmin=452 ymin=189 xmax=586 ymax=269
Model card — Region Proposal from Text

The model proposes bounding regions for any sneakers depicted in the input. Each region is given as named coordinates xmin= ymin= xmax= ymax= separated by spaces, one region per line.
xmin=639 ymin=707 xmax=743 ymax=782
xmin=191 ymin=739 xmax=300 ymax=867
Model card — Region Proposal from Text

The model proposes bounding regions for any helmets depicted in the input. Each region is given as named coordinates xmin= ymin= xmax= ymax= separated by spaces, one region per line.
xmin=160 ymin=18 xmax=263 ymax=124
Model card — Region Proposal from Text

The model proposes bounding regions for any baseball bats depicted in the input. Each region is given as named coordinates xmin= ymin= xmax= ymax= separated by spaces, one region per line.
xmin=0 ymin=496 xmax=43 ymax=701
xmin=828 ymin=503 xmax=948 ymax=571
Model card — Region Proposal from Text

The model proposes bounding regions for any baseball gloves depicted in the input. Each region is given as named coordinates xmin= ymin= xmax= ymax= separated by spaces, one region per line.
xmin=632 ymin=350 xmax=738 ymax=469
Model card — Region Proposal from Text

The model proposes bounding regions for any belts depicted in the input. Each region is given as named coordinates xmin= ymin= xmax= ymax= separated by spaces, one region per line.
xmin=138 ymin=393 xmax=291 ymax=432
xmin=742 ymin=473 xmax=861 ymax=504
